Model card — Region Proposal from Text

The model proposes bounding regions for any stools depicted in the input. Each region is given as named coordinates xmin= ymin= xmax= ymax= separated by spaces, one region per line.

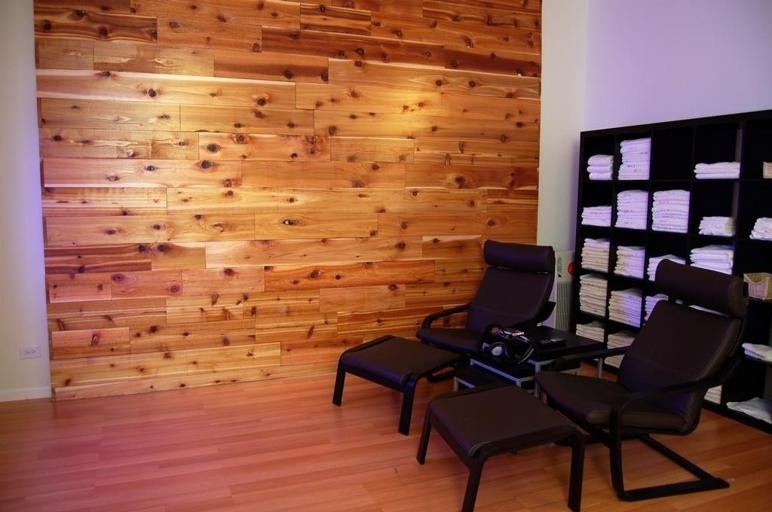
xmin=331 ymin=334 xmax=462 ymax=437
xmin=418 ymin=381 xmax=585 ymax=512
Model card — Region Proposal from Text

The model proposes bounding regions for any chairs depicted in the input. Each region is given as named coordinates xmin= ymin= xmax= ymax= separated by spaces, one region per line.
xmin=416 ymin=239 xmax=557 ymax=391
xmin=536 ymin=259 xmax=746 ymax=511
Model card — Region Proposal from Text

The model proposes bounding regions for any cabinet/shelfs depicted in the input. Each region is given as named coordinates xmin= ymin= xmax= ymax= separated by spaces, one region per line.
xmin=571 ymin=108 xmax=772 ymax=435
